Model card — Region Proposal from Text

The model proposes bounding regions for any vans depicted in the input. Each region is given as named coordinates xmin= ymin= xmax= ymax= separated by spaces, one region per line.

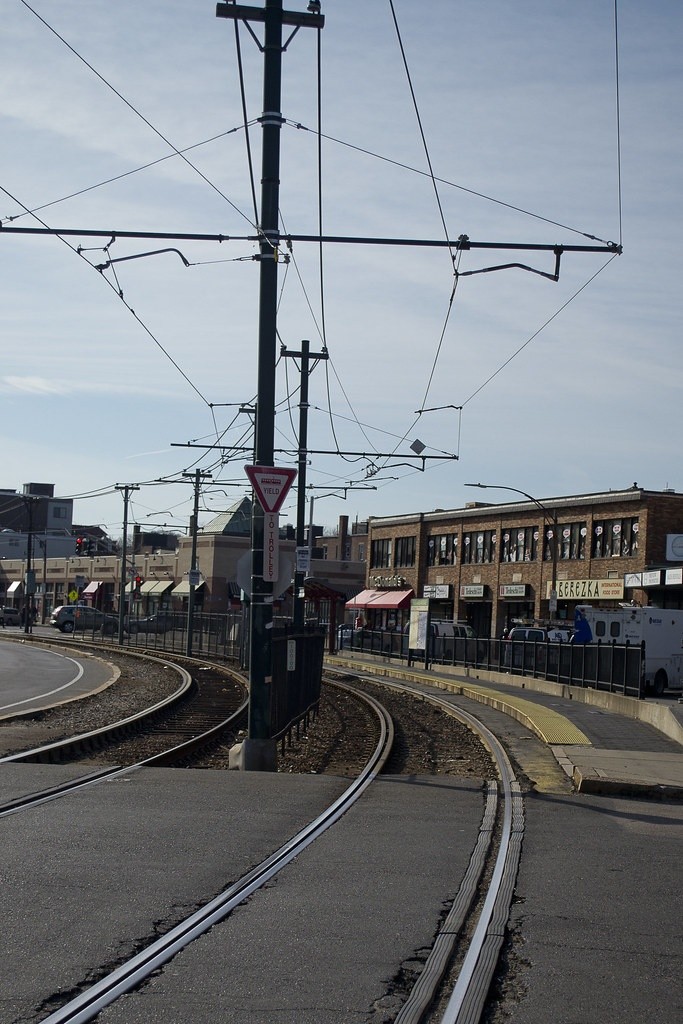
xmin=504 ymin=626 xmax=574 ymax=679
xmin=403 ymin=617 xmax=485 ymax=669
xmin=573 ymin=600 xmax=683 ymax=696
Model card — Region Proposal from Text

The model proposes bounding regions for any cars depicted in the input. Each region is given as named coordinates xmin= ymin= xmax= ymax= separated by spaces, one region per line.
xmin=0 ymin=607 xmax=34 ymax=626
xmin=337 ymin=623 xmax=356 ymax=641
xmin=125 ymin=615 xmax=172 ymax=635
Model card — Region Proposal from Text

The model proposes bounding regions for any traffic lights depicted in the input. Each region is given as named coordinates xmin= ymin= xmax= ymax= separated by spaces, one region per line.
xmin=83 ymin=538 xmax=94 ymax=555
xmin=136 ymin=575 xmax=141 ymax=593
xmin=75 ymin=537 xmax=82 ymax=555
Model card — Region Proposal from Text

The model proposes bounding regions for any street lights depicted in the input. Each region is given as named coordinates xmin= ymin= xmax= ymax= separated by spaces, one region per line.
xmin=464 ymin=482 xmax=558 ymax=626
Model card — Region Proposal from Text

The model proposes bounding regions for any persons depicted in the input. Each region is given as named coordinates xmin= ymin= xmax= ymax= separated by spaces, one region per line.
xmin=0 ymin=604 xmax=37 ymax=629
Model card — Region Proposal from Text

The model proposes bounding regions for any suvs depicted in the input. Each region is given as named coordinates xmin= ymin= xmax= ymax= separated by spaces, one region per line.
xmin=50 ymin=605 xmax=119 ymax=632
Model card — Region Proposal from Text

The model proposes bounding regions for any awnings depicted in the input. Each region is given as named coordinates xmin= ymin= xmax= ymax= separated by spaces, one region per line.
xmin=125 ymin=580 xmax=143 ymax=592
xmin=7 ymin=581 xmax=22 ymax=598
xmin=83 ymin=581 xmax=103 ymax=593
xmin=345 ymin=589 xmax=413 ymax=608
xmin=171 ymin=581 xmax=205 ymax=596
xmin=149 ymin=580 xmax=174 ymax=596
xmin=139 ymin=580 xmax=159 ymax=596
